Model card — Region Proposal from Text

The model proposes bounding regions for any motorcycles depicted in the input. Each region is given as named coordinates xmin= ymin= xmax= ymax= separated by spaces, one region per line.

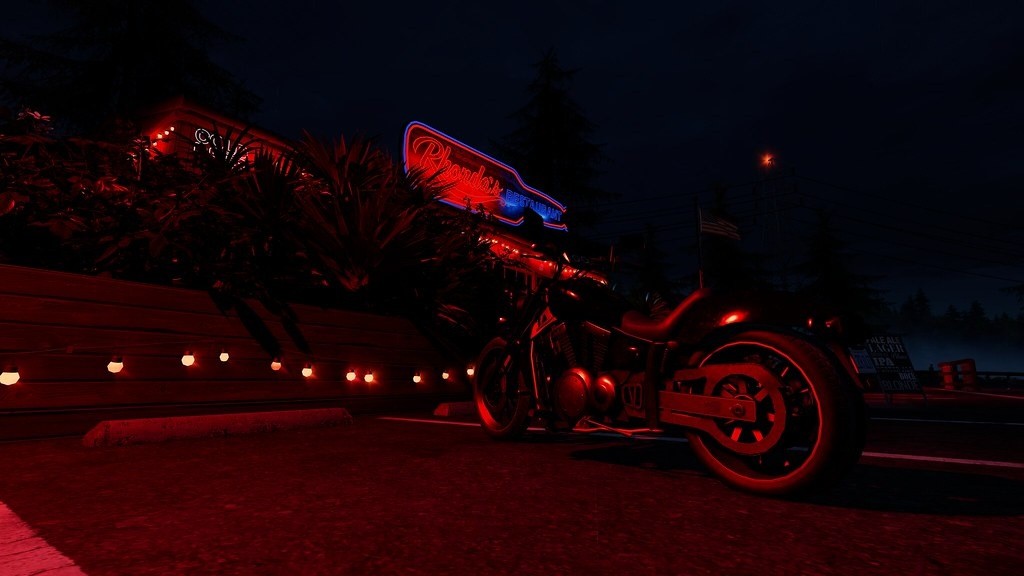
xmin=472 ymin=249 xmax=845 ymax=495
